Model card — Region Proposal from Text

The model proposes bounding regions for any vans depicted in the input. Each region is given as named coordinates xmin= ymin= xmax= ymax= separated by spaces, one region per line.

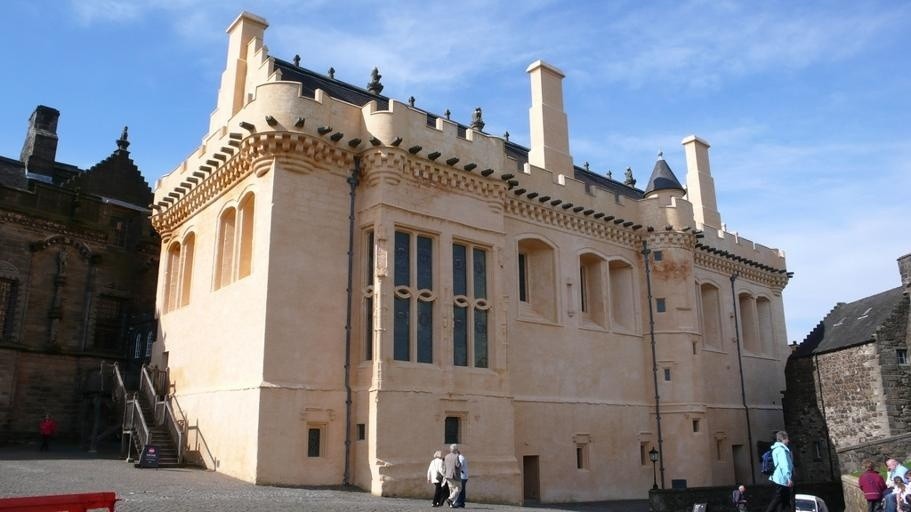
xmin=795 ymin=492 xmax=829 ymax=512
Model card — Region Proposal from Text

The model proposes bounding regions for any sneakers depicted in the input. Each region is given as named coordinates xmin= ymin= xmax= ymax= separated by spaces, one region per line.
xmin=431 ymin=497 xmax=468 ymax=508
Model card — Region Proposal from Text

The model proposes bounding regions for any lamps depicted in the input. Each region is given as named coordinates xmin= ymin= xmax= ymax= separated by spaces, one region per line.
xmin=646 ymin=445 xmax=659 ymax=489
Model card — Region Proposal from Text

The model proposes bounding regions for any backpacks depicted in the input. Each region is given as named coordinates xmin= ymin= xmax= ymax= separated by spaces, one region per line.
xmin=732 ymin=489 xmax=741 ymax=504
xmin=761 ymin=445 xmax=788 ymax=475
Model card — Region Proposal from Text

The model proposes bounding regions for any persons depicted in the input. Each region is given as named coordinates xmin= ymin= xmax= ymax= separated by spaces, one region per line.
xmin=734 ymin=485 xmax=748 ymax=512
xmin=40 ymin=414 xmax=57 ymax=449
xmin=859 ymin=457 xmax=911 ymax=512
xmin=765 ymin=430 xmax=795 ymax=512
xmin=427 ymin=444 xmax=468 ymax=508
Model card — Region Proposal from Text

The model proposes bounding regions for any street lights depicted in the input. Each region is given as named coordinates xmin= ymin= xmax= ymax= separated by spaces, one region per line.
xmin=650 ymin=447 xmax=661 ymax=491
